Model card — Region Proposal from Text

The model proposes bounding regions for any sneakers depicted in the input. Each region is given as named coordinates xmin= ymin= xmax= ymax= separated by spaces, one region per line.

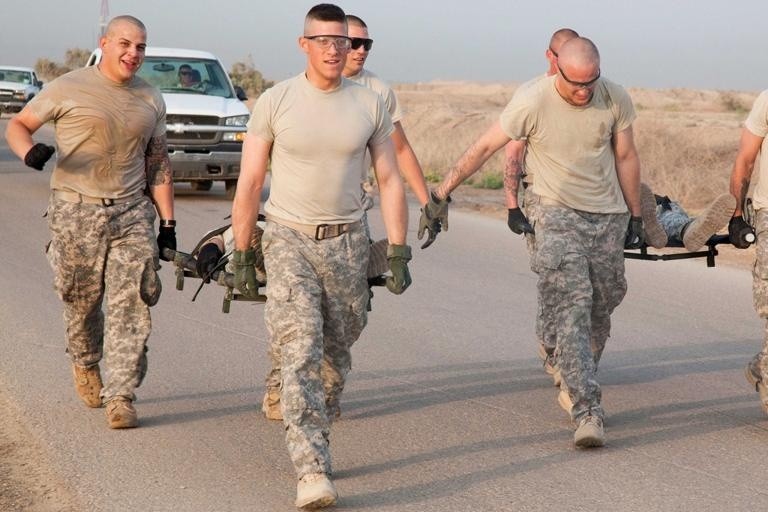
xmin=640 ymin=183 xmax=668 ymax=248
xmin=538 ymin=344 xmax=605 ymax=447
xmin=297 ymin=474 xmax=337 ymax=509
xmin=74 ymin=364 xmax=137 ymax=428
xmin=682 ymin=193 xmax=737 ymax=252
xmin=262 ymin=392 xmax=282 ymax=420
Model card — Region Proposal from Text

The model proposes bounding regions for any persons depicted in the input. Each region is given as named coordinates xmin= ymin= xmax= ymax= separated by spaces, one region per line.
xmin=502 ymin=28 xmax=580 ymax=386
xmin=6 ymin=15 xmax=175 ymax=429
xmin=635 ymin=181 xmax=739 ymax=252
xmin=178 ymin=65 xmax=197 ymax=88
xmin=232 ymin=4 xmax=411 ymax=507
xmin=260 ymin=14 xmax=437 ymax=421
xmin=425 ymin=37 xmax=645 ymax=447
xmin=731 ymin=89 xmax=768 ymax=417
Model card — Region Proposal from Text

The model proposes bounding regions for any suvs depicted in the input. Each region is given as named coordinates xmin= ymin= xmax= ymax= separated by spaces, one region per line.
xmin=81 ymin=46 xmax=255 ymax=202
xmin=0 ymin=66 xmax=44 ymax=118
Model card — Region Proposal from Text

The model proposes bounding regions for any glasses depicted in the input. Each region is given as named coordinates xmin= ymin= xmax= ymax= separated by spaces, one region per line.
xmin=180 ymin=71 xmax=192 ymax=75
xmin=559 ymin=67 xmax=600 ymax=85
xmin=305 ymin=34 xmax=372 ymax=50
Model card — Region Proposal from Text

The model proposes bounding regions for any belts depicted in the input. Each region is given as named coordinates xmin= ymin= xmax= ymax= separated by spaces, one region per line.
xmin=56 ymin=191 xmax=134 ymax=207
xmin=267 ymin=214 xmax=360 ymax=239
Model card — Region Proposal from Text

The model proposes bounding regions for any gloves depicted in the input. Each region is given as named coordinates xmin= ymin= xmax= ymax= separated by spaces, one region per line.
xmin=196 ymin=243 xmax=222 ymax=284
xmin=625 ymin=216 xmax=644 ymax=248
xmin=25 ymin=143 xmax=54 ymax=170
xmin=386 ymin=245 xmax=412 ymax=294
xmin=234 ymin=249 xmax=258 ymax=298
xmin=508 ymin=207 xmax=528 ymax=234
xmin=157 ymin=226 xmax=176 ymax=261
xmin=418 ymin=191 xmax=451 ymax=249
xmin=728 ymin=216 xmax=755 ymax=248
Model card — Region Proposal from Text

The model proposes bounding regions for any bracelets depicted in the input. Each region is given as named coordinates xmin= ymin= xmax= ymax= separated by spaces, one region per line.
xmin=160 ymin=219 xmax=176 ymax=225
